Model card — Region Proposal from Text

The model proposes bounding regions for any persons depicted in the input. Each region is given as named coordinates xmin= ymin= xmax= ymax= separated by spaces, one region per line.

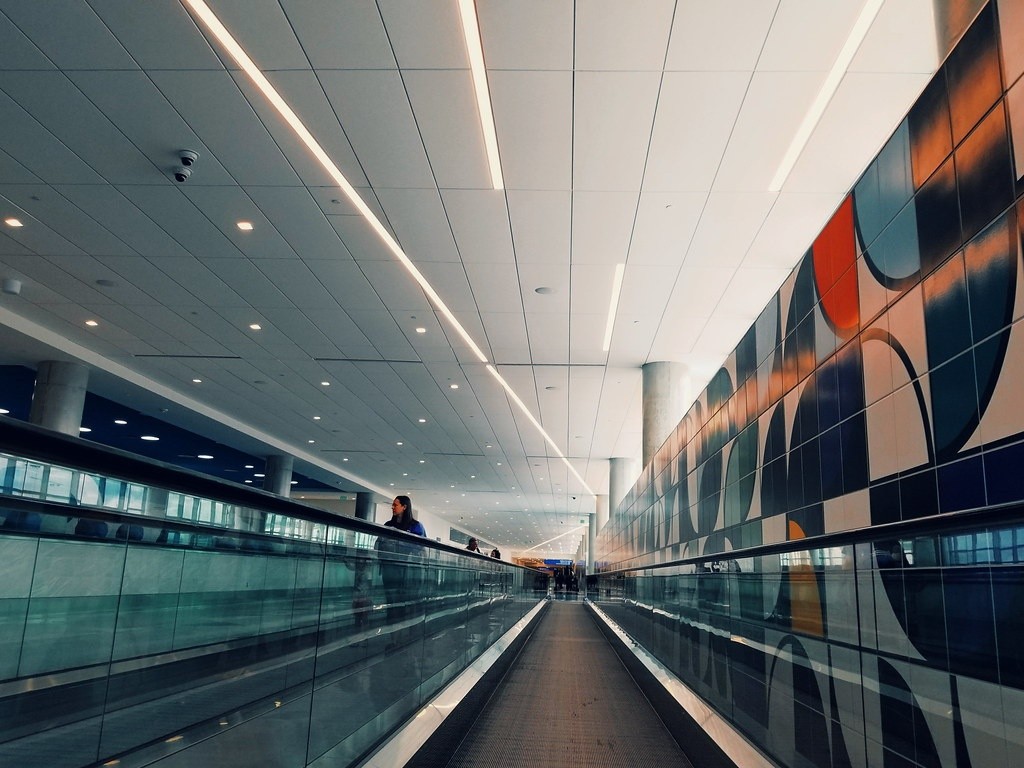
xmin=373 ymin=495 xmax=426 ymax=652
xmin=458 ymin=536 xmax=501 ymax=602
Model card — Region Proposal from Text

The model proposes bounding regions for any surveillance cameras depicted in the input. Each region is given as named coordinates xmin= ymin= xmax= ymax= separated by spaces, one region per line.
xmin=179 ymin=150 xmax=200 ymax=166
xmin=173 ymin=166 xmax=193 ymax=182
